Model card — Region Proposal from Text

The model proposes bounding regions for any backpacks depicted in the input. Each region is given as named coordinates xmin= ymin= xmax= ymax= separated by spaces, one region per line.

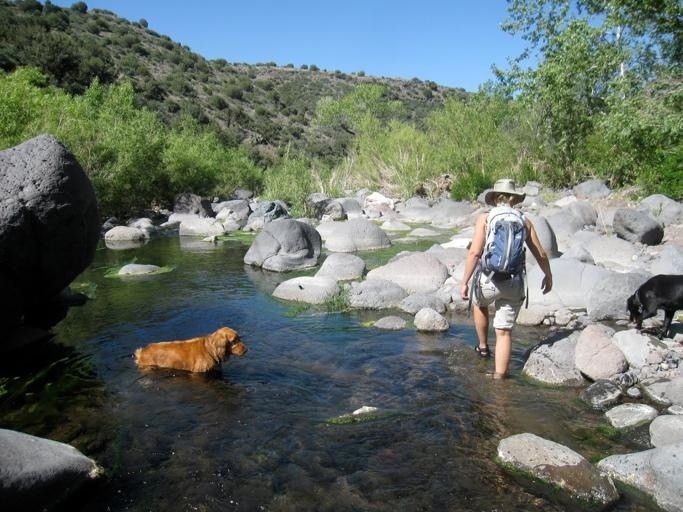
xmin=481 ymin=207 xmax=525 ymax=275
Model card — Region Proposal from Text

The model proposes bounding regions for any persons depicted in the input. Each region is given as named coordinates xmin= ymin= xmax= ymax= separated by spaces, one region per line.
xmin=458 ymin=177 xmax=554 ymax=383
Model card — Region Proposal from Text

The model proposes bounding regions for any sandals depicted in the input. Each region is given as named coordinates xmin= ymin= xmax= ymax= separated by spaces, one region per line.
xmin=474 ymin=346 xmax=489 ymax=356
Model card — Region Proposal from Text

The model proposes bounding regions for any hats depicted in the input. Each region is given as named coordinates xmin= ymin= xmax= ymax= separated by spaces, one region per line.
xmin=486 ymin=178 xmax=526 ymax=205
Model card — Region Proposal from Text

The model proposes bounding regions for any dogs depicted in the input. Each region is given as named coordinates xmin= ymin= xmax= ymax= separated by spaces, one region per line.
xmin=625 ymin=273 xmax=683 ymax=340
xmin=132 ymin=326 xmax=249 ymax=374
